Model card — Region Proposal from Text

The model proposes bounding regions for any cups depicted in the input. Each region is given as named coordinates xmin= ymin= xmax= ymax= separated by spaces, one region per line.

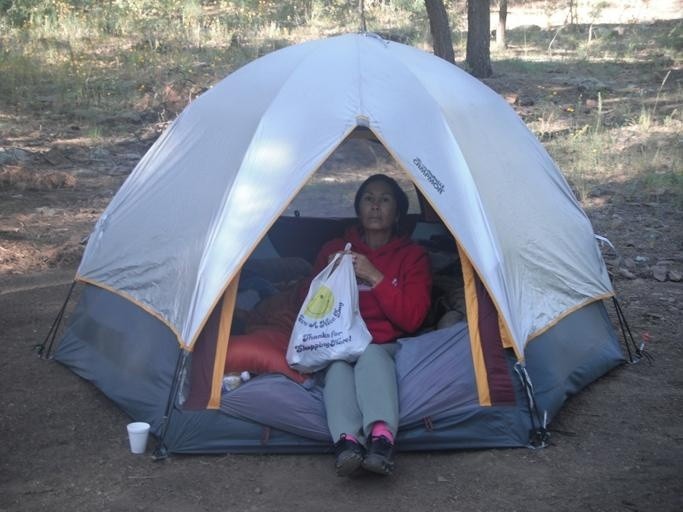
xmin=127 ymin=421 xmax=151 ymax=454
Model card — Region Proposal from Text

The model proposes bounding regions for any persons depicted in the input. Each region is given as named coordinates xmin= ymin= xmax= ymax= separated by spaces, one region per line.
xmin=295 ymin=173 xmax=432 ymax=480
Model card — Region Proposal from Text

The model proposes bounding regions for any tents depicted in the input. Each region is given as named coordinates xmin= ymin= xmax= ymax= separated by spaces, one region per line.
xmin=37 ymin=32 xmax=644 ymax=461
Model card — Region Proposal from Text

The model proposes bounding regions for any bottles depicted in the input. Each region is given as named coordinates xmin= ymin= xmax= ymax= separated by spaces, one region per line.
xmin=221 ymin=372 xmax=250 ymax=392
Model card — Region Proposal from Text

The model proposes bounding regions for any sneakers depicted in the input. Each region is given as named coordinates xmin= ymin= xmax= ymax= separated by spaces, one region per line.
xmin=363 ymin=435 xmax=394 ymax=475
xmin=329 ymin=432 xmax=378 ymax=482
